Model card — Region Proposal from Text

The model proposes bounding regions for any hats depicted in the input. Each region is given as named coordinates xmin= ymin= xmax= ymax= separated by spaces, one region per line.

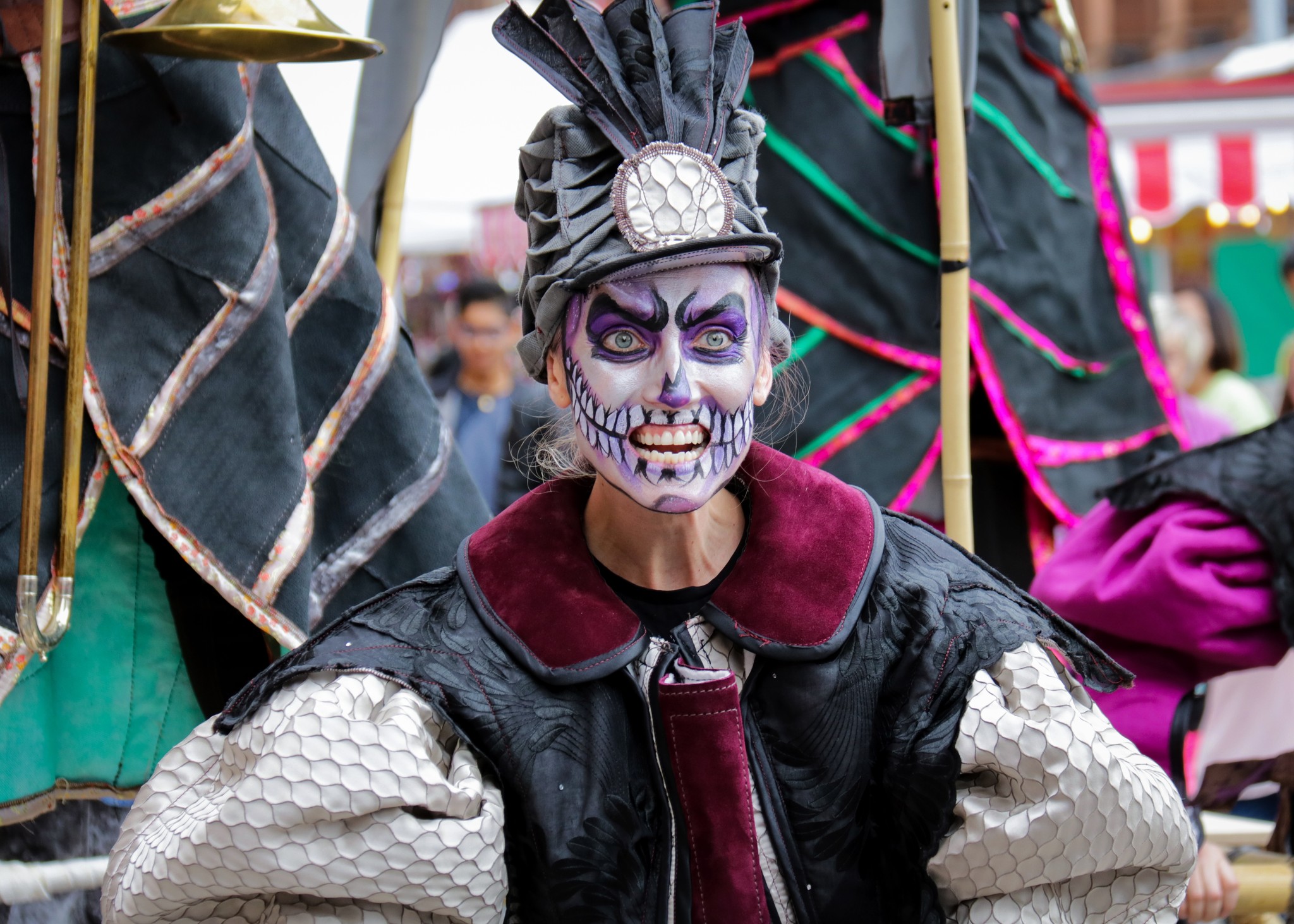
xmin=488 ymin=0 xmax=785 ymax=382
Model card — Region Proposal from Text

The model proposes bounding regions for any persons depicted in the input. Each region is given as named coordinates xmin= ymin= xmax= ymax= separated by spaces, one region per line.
xmin=412 ymin=275 xmax=580 ymax=512
xmin=95 ymin=0 xmax=1201 ymax=924
xmin=1030 ymin=238 xmax=1294 ymax=924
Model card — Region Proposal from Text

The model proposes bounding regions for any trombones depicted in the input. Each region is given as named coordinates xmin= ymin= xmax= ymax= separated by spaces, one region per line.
xmin=17 ymin=2 xmax=385 ymax=652
